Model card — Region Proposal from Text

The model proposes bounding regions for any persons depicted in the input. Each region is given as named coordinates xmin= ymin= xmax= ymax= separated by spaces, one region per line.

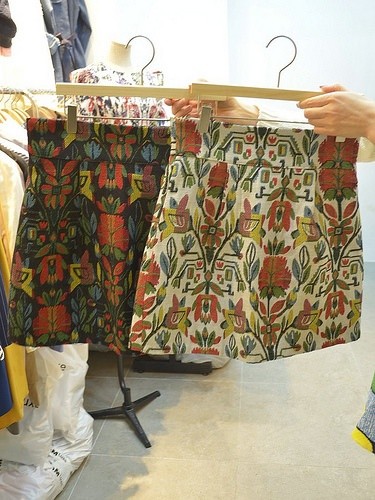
xmin=160 ymin=78 xmax=375 ymax=448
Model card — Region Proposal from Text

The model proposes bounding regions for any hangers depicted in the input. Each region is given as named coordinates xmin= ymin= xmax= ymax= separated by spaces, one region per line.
xmin=187 ymin=34 xmax=348 ymax=145
xmin=0 ymin=85 xmax=67 ymax=124
xmin=54 ymin=33 xmax=227 ymax=135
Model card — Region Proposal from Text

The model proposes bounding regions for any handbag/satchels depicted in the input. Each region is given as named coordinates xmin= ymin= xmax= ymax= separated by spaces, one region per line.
xmin=0 ymin=343 xmax=94 ymax=499
